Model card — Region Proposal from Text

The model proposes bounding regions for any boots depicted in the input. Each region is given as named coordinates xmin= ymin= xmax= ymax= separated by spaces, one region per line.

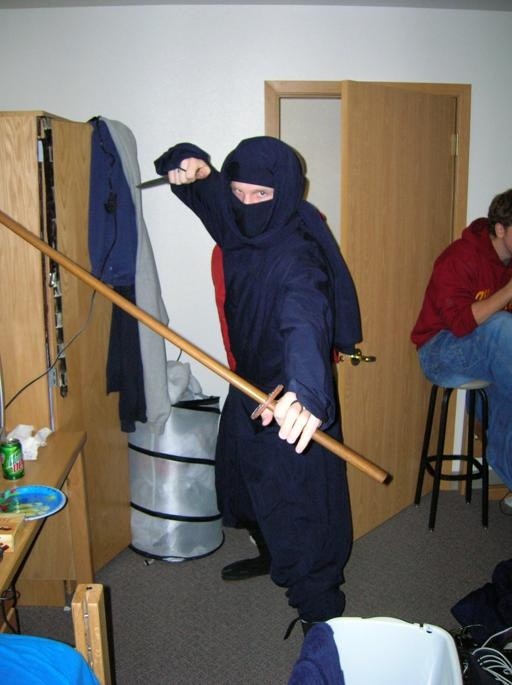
xmin=284 ymin=613 xmax=326 ymax=640
xmin=221 ymin=526 xmax=274 ymax=582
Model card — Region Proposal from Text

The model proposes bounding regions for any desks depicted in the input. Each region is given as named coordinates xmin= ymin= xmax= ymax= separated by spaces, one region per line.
xmin=0 ymin=429 xmax=93 ymax=601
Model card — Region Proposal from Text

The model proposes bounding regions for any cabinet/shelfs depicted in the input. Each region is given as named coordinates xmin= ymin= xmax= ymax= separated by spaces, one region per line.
xmin=0 ymin=111 xmax=131 ymax=606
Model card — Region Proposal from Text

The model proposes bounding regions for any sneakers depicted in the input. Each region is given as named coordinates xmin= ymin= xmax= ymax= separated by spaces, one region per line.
xmin=499 ymin=492 xmax=512 ymax=515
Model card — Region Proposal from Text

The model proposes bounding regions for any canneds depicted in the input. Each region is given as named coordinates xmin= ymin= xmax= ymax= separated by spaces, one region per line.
xmin=1 ymin=439 xmax=25 ymax=480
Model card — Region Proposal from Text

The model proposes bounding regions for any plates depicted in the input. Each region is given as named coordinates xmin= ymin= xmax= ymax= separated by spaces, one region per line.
xmin=0 ymin=485 xmax=67 ymax=520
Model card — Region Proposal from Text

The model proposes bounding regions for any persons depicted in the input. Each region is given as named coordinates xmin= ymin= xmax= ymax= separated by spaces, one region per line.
xmin=154 ymin=136 xmax=364 ymax=641
xmin=411 ymin=188 xmax=512 ymax=509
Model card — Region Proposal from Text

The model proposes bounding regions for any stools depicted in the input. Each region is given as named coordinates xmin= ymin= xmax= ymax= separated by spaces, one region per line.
xmin=413 ymin=382 xmax=491 ymax=532
